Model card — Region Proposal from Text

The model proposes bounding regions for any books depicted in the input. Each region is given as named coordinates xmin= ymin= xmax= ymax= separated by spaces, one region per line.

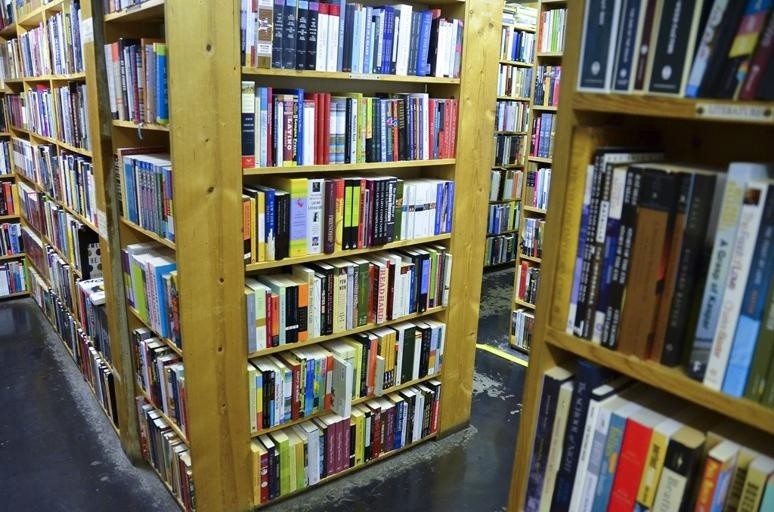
xmin=106 ymin=0 xmax=196 ymax=511
xmin=241 ymin=0 xmax=463 ymax=505
xmin=524 ymin=0 xmax=774 ymax=511
xmin=1 ymin=0 xmax=117 ymax=428
xmin=484 ymin=2 xmax=567 ymax=354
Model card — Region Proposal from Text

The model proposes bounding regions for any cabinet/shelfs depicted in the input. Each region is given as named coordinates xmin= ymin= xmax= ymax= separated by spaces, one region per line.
xmin=506 ymin=4 xmax=773 ymax=510
xmin=0 ymin=0 xmax=539 ymax=512
